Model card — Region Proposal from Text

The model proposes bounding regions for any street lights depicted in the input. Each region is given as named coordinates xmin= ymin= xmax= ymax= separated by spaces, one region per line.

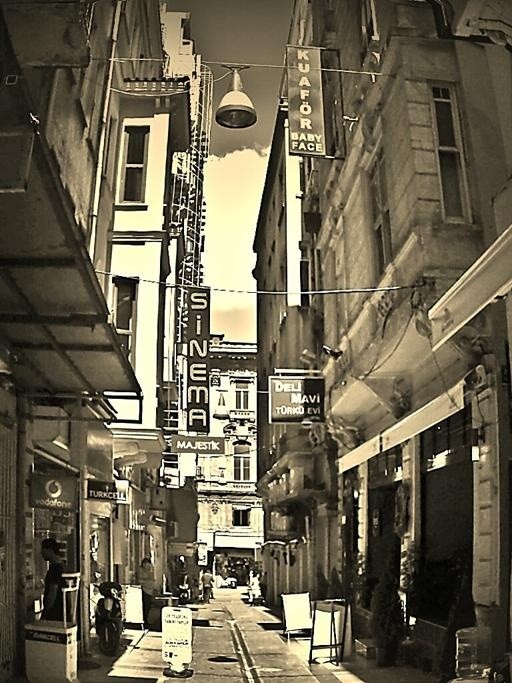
xmin=212 ymin=530 xmax=230 ymax=582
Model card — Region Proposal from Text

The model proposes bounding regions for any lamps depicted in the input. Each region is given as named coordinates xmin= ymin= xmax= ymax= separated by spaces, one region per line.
xmin=214 ymin=65 xmax=259 ymax=130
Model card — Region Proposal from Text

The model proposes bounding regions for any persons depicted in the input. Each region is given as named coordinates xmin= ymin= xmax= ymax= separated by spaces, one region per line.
xmin=41 ymin=537 xmax=72 ymax=622
xmin=136 ymin=558 xmax=155 ymax=630
xmin=201 ymin=569 xmax=215 ymax=598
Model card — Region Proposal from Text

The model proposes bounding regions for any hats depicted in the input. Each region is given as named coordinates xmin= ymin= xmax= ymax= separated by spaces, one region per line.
xmin=43 ymin=537 xmax=64 ymax=554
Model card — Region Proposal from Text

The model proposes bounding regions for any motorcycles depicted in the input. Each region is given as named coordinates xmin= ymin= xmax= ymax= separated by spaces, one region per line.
xmin=93 ymin=582 xmax=128 ymax=657
xmin=178 ymin=584 xmax=188 ymax=604
xmin=204 ymin=584 xmax=211 ymax=602
